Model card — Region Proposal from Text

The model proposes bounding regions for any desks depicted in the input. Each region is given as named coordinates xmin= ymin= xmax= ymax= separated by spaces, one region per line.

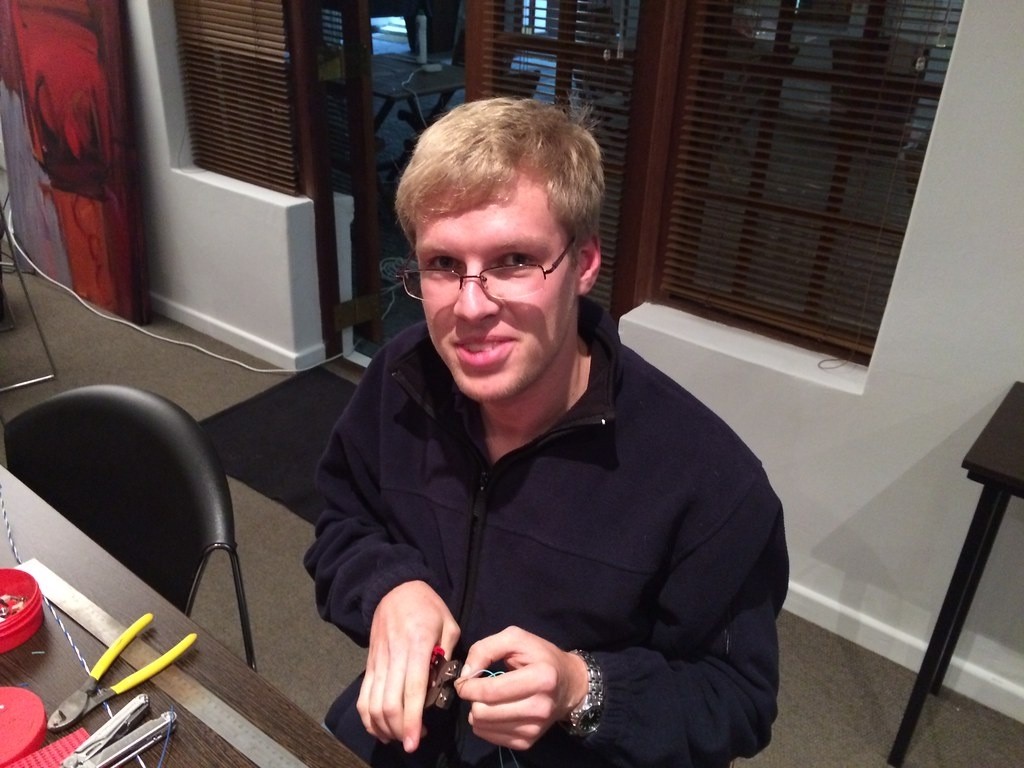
xmin=886 ymin=381 xmax=1024 ymax=768
xmin=372 ymin=51 xmax=466 ymax=181
xmin=0 ymin=464 xmax=369 ymax=768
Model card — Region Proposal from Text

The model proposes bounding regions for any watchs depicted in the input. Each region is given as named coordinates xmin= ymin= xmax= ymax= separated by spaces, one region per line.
xmin=558 ymin=648 xmax=604 ymax=736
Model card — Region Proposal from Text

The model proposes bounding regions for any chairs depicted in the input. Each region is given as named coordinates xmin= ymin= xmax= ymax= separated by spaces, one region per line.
xmin=3 ymin=384 xmax=257 ymax=674
xmin=397 ymin=70 xmax=541 ymax=135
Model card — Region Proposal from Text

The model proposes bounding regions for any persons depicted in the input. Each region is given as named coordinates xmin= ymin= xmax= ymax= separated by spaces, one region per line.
xmin=303 ymin=98 xmax=790 ymax=768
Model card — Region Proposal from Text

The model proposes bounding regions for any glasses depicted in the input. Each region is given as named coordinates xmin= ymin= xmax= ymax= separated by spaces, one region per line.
xmin=403 ymin=235 xmax=579 ymax=299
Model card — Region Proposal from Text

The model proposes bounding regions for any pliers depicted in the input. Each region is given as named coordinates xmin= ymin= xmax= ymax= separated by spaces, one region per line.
xmin=47 ymin=612 xmax=198 ymax=732
xmin=60 ymin=692 xmax=183 ymax=768
xmin=424 ymin=645 xmax=464 ymax=711
